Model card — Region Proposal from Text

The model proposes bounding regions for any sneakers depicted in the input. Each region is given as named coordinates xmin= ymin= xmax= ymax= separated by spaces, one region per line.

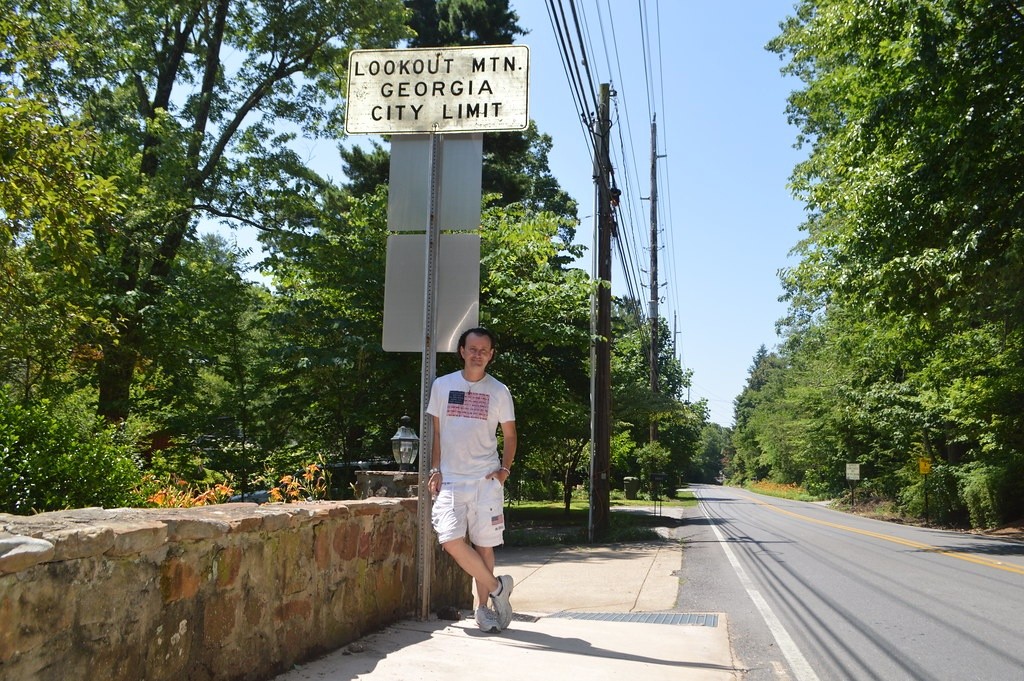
xmin=489 ymin=574 xmax=514 ymax=630
xmin=474 ymin=605 xmax=501 ymax=633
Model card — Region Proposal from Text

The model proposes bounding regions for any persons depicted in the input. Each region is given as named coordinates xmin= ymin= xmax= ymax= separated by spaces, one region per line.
xmin=426 ymin=328 xmax=517 ymax=633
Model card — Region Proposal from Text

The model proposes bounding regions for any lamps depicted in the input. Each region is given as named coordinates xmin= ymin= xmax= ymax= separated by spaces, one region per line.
xmin=390 ymin=408 xmax=420 ymax=471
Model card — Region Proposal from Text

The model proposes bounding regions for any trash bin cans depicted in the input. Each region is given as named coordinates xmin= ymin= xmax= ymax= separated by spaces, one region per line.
xmin=623 ymin=476 xmax=641 ymax=500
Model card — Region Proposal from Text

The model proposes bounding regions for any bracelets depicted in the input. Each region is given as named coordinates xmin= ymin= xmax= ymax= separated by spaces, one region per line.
xmin=428 ymin=468 xmax=440 ymax=476
xmin=500 ymin=467 xmax=511 ymax=476
